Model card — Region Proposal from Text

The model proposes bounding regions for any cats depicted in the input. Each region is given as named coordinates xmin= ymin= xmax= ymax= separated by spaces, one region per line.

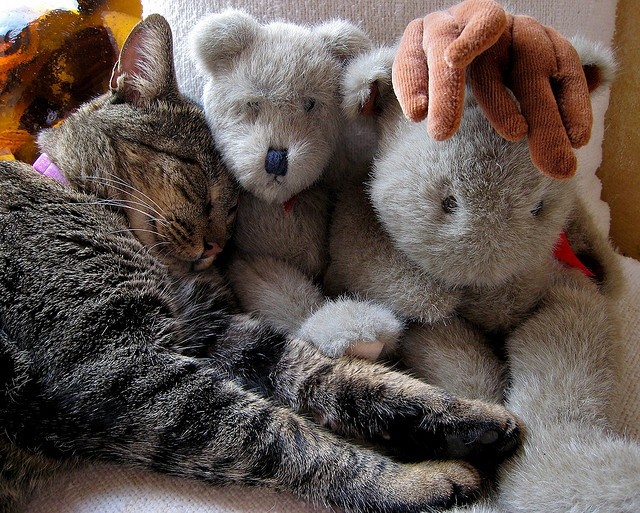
xmin=1 ymin=12 xmax=525 ymax=512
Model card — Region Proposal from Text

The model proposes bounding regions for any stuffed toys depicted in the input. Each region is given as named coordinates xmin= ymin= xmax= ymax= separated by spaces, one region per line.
xmin=340 ymin=0 xmax=640 ymax=513
xmin=187 ymin=9 xmax=405 ymax=359
